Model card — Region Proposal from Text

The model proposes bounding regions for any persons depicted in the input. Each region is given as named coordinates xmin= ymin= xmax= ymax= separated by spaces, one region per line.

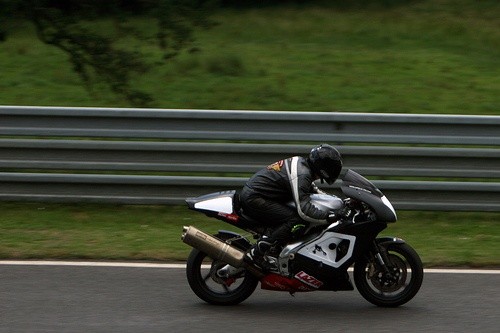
xmin=239 ymin=143 xmax=354 ymax=274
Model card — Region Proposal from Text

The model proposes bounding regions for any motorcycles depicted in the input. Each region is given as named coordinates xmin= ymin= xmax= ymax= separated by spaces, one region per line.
xmin=178 ymin=169 xmax=424 ymax=309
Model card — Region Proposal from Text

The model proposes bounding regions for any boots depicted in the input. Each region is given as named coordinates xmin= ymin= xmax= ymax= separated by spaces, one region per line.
xmin=248 ymin=231 xmax=276 ymax=274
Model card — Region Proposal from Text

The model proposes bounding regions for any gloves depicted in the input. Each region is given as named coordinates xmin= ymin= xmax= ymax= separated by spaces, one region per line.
xmin=326 ymin=205 xmax=353 ymax=223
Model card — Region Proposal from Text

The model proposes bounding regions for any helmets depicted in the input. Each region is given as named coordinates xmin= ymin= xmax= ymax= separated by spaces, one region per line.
xmin=307 ymin=144 xmax=343 ymax=185
